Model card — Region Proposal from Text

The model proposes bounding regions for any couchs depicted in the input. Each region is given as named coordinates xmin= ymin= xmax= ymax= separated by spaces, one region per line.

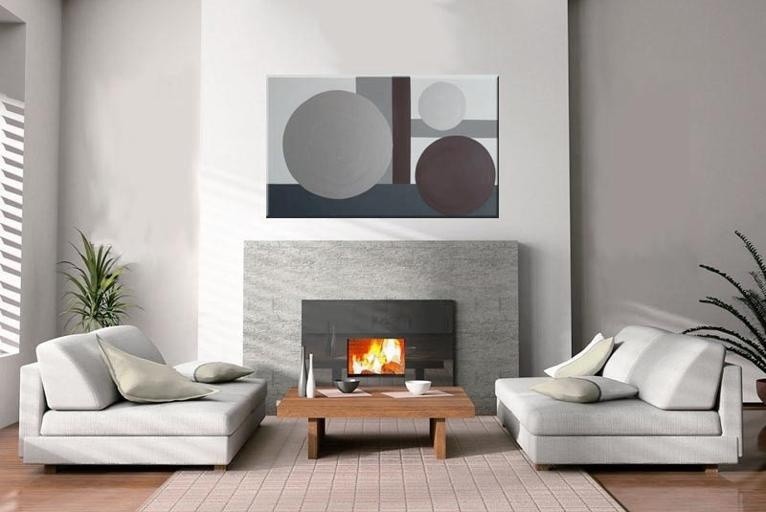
xmin=493 ymin=321 xmax=744 ymax=476
xmin=14 ymin=323 xmax=269 ymax=472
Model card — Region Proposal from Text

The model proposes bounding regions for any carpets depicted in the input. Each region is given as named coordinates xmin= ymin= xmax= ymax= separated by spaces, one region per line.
xmin=133 ymin=407 xmax=636 ymax=511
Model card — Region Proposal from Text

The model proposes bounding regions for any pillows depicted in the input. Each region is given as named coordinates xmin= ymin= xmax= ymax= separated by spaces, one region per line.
xmin=173 ymin=357 xmax=256 ymax=385
xmin=541 ymin=331 xmax=617 ymax=378
xmin=94 ymin=332 xmax=219 ymax=403
xmin=528 ymin=374 xmax=640 ymax=403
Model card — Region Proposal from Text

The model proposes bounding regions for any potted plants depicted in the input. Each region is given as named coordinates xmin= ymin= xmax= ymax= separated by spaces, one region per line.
xmin=678 ymin=224 xmax=765 ymax=411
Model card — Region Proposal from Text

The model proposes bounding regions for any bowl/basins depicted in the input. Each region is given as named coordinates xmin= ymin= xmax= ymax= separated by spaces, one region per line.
xmin=405 ymin=380 xmax=432 ymax=395
xmin=333 ymin=379 xmax=361 ymax=392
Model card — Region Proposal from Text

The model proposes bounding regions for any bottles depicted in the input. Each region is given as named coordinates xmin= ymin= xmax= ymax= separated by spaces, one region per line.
xmin=298 ymin=346 xmax=307 ymax=398
xmin=305 ymin=353 xmax=316 ymax=398
